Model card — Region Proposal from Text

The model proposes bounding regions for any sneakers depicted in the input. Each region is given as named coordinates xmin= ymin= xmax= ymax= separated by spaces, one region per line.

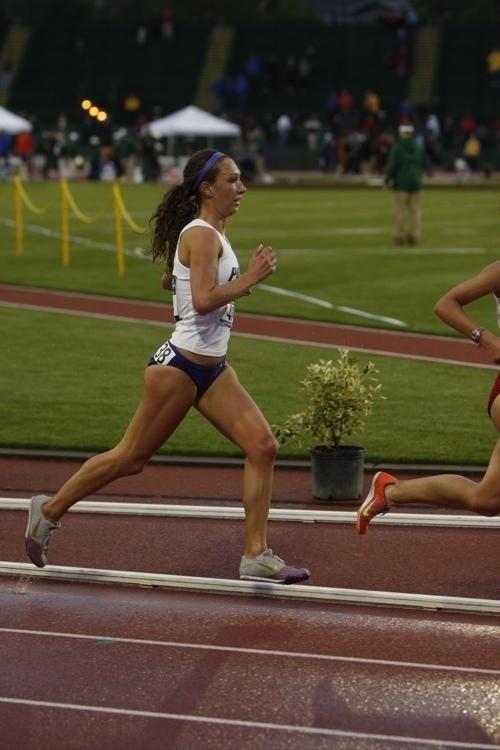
xmin=357 ymin=471 xmax=398 ymax=535
xmin=239 ymin=548 xmax=310 ymax=583
xmin=25 ymin=495 xmax=61 ymax=568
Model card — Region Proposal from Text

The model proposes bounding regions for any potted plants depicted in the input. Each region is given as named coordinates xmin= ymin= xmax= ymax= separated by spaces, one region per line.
xmin=272 ymin=346 xmax=388 ymax=501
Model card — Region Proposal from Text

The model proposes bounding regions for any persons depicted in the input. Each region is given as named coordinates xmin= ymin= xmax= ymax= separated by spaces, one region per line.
xmin=25 ymin=147 xmax=310 ymax=585
xmin=0 ymin=129 xmax=163 ymax=184
xmin=384 ymin=123 xmax=425 ymax=244
xmin=217 ymin=88 xmax=499 ymax=183
xmin=357 ymin=260 xmax=500 ymax=534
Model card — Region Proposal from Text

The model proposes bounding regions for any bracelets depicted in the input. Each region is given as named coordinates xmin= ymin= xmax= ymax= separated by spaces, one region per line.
xmin=471 ymin=326 xmax=485 ymax=343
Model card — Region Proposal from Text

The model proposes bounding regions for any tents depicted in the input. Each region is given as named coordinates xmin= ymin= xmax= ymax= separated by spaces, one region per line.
xmin=136 ymin=104 xmax=242 ymax=153
xmin=1 ymin=108 xmax=33 ymax=132
